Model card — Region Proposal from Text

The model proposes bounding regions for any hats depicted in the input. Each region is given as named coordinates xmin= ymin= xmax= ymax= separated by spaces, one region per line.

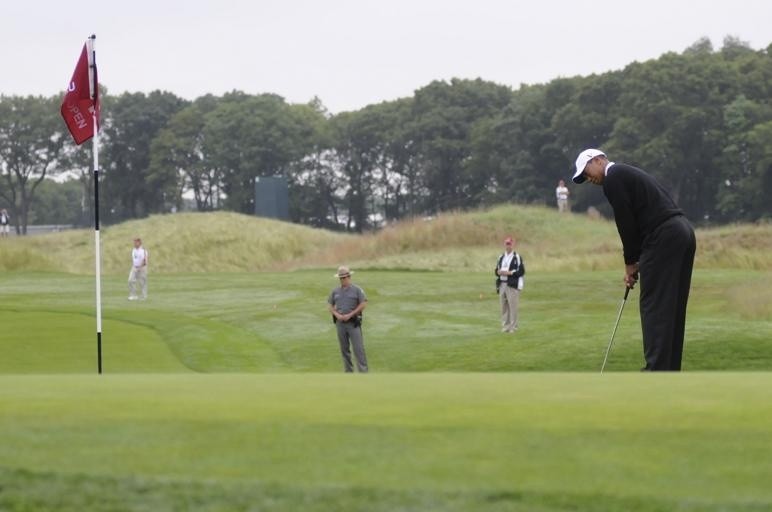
xmin=572 ymin=148 xmax=607 ymax=185
xmin=335 ymin=266 xmax=354 ymax=278
xmin=504 ymin=237 xmax=513 ymax=244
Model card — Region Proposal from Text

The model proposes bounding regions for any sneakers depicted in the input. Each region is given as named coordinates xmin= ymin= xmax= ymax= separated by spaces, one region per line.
xmin=128 ymin=296 xmax=146 ymax=300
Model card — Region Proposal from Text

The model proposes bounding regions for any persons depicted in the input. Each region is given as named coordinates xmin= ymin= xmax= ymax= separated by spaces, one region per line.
xmin=494 ymin=236 xmax=525 ymax=333
xmin=0 ymin=209 xmax=10 ymax=240
xmin=128 ymin=239 xmax=148 ymax=301
xmin=572 ymin=149 xmax=696 ymax=372
xmin=555 ymin=179 xmax=569 ymax=213
xmin=327 ymin=266 xmax=369 ymax=373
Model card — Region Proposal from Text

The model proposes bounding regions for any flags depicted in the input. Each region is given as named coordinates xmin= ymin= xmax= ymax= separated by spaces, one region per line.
xmin=61 ymin=41 xmax=101 ymax=145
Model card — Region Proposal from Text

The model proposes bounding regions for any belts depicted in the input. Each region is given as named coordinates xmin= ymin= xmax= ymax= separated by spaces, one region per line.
xmin=499 ymin=280 xmax=510 ymax=282
